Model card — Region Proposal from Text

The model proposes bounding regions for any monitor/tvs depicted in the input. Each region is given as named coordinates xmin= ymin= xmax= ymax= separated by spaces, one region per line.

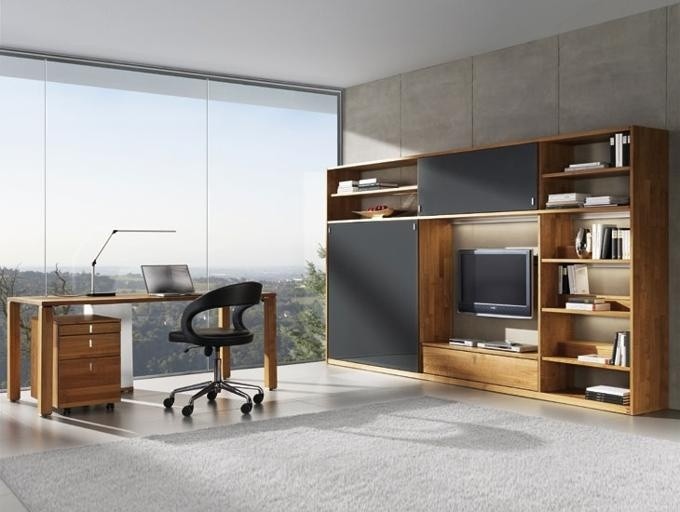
xmin=457 ymin=248 xmax=531 ymax=316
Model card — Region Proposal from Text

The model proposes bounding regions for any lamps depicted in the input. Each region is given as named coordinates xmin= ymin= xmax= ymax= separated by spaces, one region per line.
xmin=85 ymin=227 xmax=178 ymax=298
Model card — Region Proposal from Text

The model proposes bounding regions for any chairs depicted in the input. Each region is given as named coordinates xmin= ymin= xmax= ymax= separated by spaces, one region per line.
xmin=158 ymin=276 xmax=269 ymax=418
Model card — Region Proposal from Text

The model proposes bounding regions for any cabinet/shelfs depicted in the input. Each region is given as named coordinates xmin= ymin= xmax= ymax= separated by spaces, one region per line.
xmin=28 ymin=311 xmax=125 ymax=418
xmin=536 ymin=121 xmax=670 ymax=416
xmin=416 ymin=137 xmax=577 ymax=403
xmin=323 ymin=155 xmax=457 ymax=380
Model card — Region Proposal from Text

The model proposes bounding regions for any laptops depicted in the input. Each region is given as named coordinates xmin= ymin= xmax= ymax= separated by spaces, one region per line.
xmin=141 ymin=264 xmax=204 ymax=297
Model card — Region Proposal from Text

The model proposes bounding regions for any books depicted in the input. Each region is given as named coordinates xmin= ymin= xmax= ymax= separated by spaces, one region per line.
xmin=545 ymin=132 xmax=631 ymax=404
xmin=337 ymin=177 xmax=398 ymax=195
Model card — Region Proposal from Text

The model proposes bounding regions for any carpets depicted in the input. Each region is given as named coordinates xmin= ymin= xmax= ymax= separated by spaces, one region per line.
xmin=1 ymin=393 xmax=679 ymax=511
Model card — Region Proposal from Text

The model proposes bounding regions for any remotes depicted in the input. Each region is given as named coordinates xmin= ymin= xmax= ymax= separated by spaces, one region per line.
xmin=505 ymin=339 xmax=516 ymax=346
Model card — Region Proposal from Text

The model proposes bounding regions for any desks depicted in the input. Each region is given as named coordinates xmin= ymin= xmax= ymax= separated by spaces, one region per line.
xmin=2 ymin=288 xmax=281 ymax=420
